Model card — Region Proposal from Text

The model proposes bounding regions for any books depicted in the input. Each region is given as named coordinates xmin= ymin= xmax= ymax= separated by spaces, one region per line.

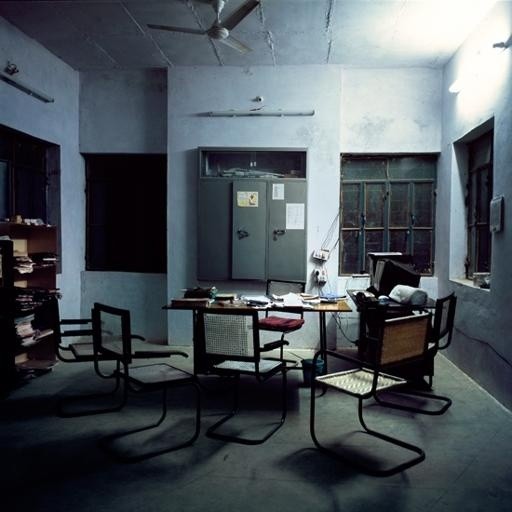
xmin=0 ymin=233 xmax=64 ymax=372
xmin=170 ymin=284 xmax=349 ymax=309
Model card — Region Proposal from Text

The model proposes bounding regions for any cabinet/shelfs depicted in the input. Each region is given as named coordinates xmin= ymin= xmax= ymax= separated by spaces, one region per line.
xmin=196 ymin=146 xmax=308 ymax=284
xmin=0 ymin=222 xmax=61 ymax=391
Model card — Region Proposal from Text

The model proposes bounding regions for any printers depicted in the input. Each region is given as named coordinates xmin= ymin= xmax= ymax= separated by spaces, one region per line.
xmin=389 ymin=284 xmax=428 ymax=305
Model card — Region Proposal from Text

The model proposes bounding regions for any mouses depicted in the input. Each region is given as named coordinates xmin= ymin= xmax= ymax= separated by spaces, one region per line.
xmin=377 ymin=295 xmax=389 ymax=300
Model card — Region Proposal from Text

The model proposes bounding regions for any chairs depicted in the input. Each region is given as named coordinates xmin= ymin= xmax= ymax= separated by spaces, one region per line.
xmin=198 ymin=307 xmax=288 ymax=444
xmin=50 ymin=292 xmax=127 ymax=419
xmin=310 ymin=312 xmax=431 ymax=478
xmin=372 ymin=292 xmax=456 ymax=415
xmin=90 ymin=302 xmax=203 ymax=460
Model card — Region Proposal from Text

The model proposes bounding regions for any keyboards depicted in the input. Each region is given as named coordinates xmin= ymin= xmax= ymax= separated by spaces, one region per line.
xmin=353 ymin=290 xmax=375 ymax=298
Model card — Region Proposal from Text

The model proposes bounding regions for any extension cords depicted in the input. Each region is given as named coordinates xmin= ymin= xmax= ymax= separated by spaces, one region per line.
xmin=314 ymin=269 xmax=327 ymax=283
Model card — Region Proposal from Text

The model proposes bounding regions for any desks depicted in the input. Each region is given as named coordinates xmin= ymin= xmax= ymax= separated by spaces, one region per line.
xmin=162 ymin=292 xmax=350 ymax=376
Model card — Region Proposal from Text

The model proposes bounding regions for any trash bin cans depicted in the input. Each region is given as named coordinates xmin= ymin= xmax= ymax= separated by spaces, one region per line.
xmin=301 ymin=359 xmax=324 ymax=387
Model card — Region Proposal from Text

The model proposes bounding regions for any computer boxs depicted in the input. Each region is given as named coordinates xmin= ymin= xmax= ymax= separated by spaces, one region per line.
xmin=367 ymin=252 xmax=404 ymax=287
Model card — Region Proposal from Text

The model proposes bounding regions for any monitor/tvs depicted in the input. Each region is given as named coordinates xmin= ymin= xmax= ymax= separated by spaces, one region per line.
xmin=373 ymin=258 xmax=421 ymax=296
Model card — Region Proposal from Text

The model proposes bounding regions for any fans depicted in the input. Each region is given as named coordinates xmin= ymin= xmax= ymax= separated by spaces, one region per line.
xmin=147 ymin=0 xmax=260 ymax=56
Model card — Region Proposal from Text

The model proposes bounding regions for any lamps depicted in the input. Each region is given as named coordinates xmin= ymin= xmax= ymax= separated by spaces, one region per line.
xmin=0 ymin=68 xmax=55 ymax=104
xmin=209 ymin=109 xmax=314 ymax=118
xmin=449 ymin=42 xmax=506 ymax=93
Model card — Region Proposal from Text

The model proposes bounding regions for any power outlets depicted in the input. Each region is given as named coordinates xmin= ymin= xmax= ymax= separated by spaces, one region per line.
xmin=314 ymin=269 xmax=327 ymax=282
